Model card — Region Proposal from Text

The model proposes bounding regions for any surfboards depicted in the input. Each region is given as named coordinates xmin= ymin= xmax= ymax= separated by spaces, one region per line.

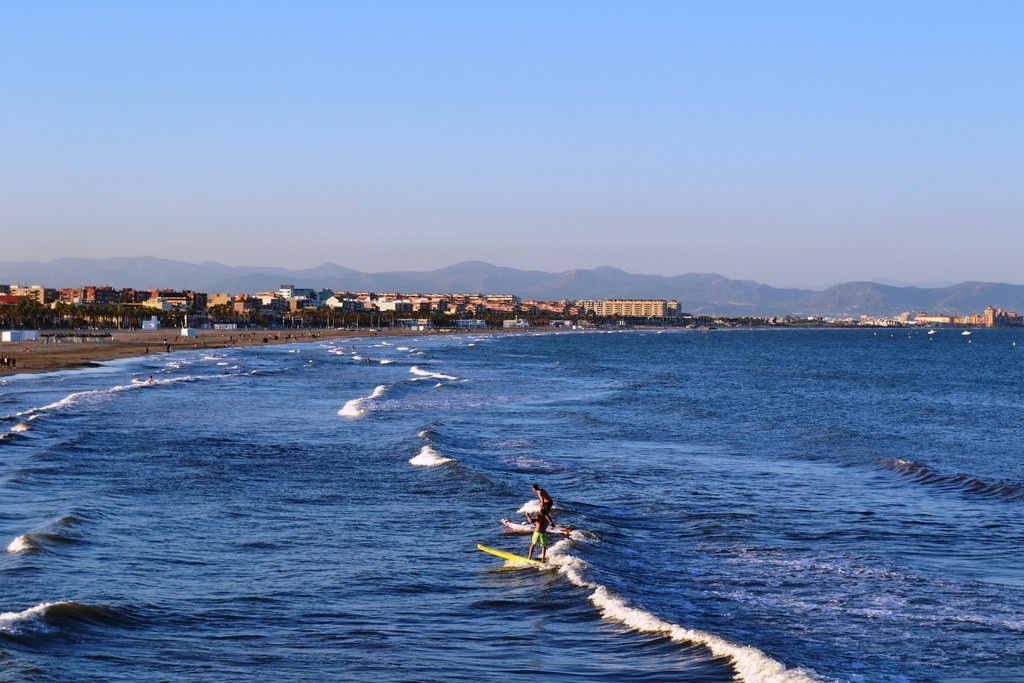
xmin=476 ymin=543 xmax=545 ymax=565
xmin=500 ymin=518 xmax=571 ymax=535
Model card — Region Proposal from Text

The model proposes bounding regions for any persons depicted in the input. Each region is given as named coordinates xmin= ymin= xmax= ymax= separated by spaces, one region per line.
xmin=117 ymin=321 xmax=534 ymax=356
xmin=0 ymin=353 xmax=18 ymax=368
xmin=532 ymin=483 xmax=554 ymax=528
xmin=148 ymin=375 xmax=154 ymax=384
xmin=524 ymin=507 xmax=552 ymax=563
